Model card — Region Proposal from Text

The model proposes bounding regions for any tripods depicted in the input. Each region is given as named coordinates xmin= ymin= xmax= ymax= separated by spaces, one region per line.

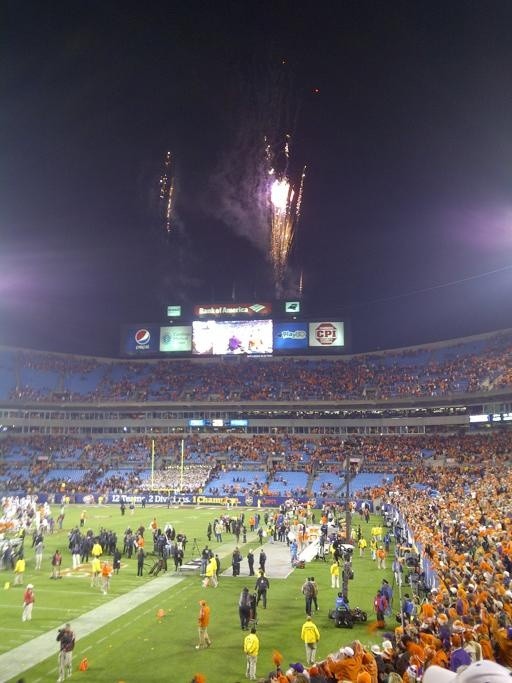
xmin=190 ymin=537 xmax=201 ymax=558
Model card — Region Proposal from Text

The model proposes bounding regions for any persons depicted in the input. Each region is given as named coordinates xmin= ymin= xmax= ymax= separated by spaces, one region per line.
xmin=1 ymin=327 xmax=512 ymax=683
